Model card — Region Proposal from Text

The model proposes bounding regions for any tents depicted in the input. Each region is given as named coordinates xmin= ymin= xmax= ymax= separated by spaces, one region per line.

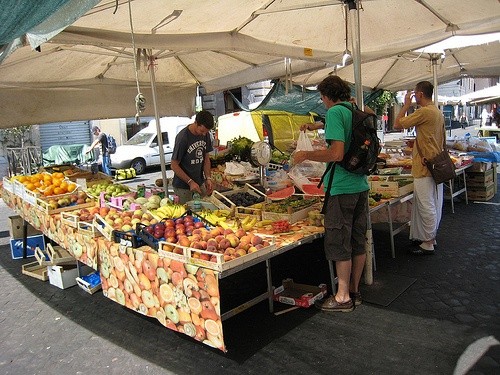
xmin=0 ymin=0 xmax=500 ymax=287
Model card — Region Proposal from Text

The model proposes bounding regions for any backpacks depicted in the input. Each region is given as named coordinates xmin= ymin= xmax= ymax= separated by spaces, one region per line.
xmin=99 ymin=133 xmax=116 ymax=154
xmin=326 ymin=102 xmax=379 ymax=174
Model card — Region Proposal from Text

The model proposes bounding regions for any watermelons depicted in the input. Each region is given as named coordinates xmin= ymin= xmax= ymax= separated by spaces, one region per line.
xmin=301 ymin=184 xmax=325 ymax=195
xmin=267 ymin=185 xmax=295 ymax=200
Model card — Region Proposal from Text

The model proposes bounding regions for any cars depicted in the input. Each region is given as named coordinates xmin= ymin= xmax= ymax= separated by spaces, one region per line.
xmin=99 ymin=118 xmax=193 ymax=176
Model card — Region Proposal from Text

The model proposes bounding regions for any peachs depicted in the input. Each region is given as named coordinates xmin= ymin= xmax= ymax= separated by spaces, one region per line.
xmin=143 ymin=215 xmax=271 ymax=265
xmin=72 ymin=206 xmax=157 ymax=234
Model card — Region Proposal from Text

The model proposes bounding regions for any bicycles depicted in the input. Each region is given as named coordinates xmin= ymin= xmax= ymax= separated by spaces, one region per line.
xmin=71 ymin=152 xmax=102 ymax=172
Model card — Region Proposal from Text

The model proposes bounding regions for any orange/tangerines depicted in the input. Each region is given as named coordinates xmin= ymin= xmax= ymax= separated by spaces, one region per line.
xmin=99 ymin=241 xmax=224 ymax=350
xmin=23 ymin=202 xmax=98 ymax=271
xmin=12 ymin=172 xmax=77 ymax=196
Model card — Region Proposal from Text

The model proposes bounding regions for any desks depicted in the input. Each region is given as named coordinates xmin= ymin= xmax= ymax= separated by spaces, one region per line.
xmin=0 ymin=155 xmax=470 ymax=351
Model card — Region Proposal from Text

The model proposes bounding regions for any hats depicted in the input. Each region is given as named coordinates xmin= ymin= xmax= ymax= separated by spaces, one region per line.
xmin=91 ymin=126 xmax=98 ymax=134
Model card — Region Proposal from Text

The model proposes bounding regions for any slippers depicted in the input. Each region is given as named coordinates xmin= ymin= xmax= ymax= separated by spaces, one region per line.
xmin=417 ymin=242 xmax=438 ymax=248
xmin=407 ymin=244 xmax=434 ymax=255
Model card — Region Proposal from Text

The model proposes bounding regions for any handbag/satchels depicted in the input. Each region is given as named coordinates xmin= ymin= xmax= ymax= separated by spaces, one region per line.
xmin=296 ymin=130 xmax=313 ymax=152
xmin=425 ymin=151 xmax=456 ymax=183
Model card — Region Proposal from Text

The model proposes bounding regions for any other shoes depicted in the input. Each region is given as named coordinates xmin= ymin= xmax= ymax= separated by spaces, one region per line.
xmin=316 ymin=295 xmax=354 ymax=312
xmin=349 ymin=292 xmax=362 ymax=305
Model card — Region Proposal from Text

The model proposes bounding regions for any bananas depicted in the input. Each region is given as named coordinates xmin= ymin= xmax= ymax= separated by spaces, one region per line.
xmin=200 ymin=208 xmax=270 ymax=231
xmin=150 ymin=204 xmax=188 ymax=219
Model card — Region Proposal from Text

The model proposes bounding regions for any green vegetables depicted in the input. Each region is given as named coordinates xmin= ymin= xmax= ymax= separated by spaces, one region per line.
xmin=227 ymin=135 xmax=254 ymax=155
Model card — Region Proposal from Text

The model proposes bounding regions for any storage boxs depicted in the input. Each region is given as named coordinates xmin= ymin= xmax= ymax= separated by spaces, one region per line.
xmin=47 ymin=261 xmax=95 ymax=289
xmin=262 ymin=201 xmax=320 ymax=225
xmin=9 ymin=235 xmax=44 ymax=260
xmin=9 ymin=215 xmax=42 ymax=238
xmin=467 ymin=161 xmax=498 ymax=201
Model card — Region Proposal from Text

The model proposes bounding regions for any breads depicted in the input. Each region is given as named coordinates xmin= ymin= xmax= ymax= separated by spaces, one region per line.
xmin=378 ymin=153 xmax=412 ymax=165
xmin=454 ymin=140 xmax=489 ymax=152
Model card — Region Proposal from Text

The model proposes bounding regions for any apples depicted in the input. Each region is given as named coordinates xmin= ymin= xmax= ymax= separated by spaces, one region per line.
xmin=41 ymin=191 xmax=91 ymax=209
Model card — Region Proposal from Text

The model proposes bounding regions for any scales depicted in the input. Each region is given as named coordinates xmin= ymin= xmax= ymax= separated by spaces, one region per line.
xmin=250 ymin=140 xmax=273 ymax=192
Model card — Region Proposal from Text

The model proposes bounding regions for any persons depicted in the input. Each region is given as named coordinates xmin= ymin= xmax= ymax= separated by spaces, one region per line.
xmin=293 ymin=75 xmax=382 ymax=312
xmin=170 ymin=111 xmax=214 ymax=206
xmin=86 ymin=126 xmax=112 ymax=176
xmin=394 ymin=81 xmax=445 ymax=255
xmin=489 ymin=109 xmax=494 ymax=124
xmin=459 ymin=113 xmax=469 ymax=129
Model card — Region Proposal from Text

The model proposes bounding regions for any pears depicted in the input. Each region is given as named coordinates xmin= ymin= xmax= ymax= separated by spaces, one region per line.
xmin=86 ymin=183 xmax=130 ymax=200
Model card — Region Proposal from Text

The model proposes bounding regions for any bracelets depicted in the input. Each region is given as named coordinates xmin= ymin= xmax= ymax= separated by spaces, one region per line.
xmin=187 ymin=178 xmax=192 ymax=185
xmin=204 ymin=177 xmax=211 ymax=180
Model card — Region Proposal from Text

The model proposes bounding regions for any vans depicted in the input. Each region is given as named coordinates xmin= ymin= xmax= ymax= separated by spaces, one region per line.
xmin=217 ymin=108 xmax=322 ymax=159
